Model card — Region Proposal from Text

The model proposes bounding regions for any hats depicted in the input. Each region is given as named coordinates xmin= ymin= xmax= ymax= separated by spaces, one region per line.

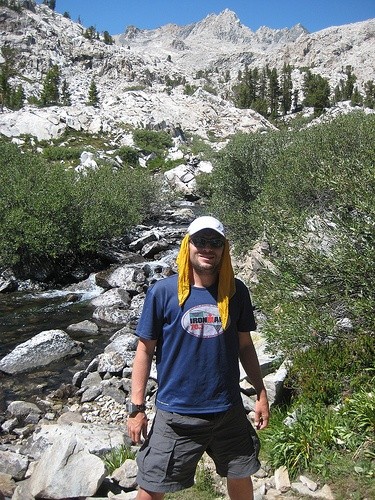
xmin=187 ymin=216 xmax=226 ymax=238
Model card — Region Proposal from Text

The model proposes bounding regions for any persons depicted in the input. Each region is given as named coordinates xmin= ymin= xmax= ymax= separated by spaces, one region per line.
xmin=127 ymin=216 xmax=271 ymax=500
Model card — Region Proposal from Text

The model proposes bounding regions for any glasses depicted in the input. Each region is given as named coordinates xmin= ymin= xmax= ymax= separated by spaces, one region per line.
xmin=188 ymin=237 xmax=225 ymax=248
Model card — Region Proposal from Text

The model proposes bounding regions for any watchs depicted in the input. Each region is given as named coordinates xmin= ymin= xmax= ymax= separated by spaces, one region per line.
xmin=125 ymin=402 xmax=147 ymax=415
